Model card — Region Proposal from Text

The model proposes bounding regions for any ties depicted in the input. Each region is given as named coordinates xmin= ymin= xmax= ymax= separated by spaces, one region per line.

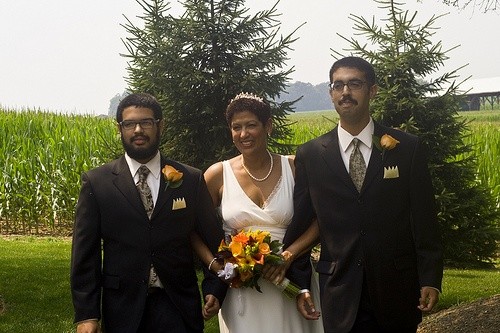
xmin=349 ymin=138 xmax=368 ymax=194
xmin=135 ymin=164 xmax=157 ymax=288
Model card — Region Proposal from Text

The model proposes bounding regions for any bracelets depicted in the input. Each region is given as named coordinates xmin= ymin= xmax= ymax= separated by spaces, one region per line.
xmin=280 ymin=251 xmax=293 ymax=263
xmin=208 ymin=257 xmax=217 ymax=270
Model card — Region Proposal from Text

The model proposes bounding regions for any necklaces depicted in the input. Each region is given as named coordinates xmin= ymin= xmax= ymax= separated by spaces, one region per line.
xmin=241 ymin=148 xmax=273 ymax=182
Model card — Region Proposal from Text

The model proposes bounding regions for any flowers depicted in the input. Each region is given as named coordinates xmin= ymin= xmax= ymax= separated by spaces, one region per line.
xmin=372 ymin=132 xmax=400 ymax=160
xmin=219 ymin=227 xmax=302 ymax=299
xmin=162 ymin=165 xmax=184 ymax=193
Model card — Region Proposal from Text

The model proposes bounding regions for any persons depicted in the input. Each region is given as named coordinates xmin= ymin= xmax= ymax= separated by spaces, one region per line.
xmin=192 ymin=91 xmax=325 ymax=333
xmin=70 ymin=93 xmax=228 ymax=333
xmin=282 ymin=57 xmax=444 ymax=333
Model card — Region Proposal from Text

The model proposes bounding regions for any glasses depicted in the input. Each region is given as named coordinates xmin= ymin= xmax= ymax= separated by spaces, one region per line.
xmin=119 ymin=119 xmax=160 ymax=130
xmin=330 ymin=79 xmax=370 ymax=90
